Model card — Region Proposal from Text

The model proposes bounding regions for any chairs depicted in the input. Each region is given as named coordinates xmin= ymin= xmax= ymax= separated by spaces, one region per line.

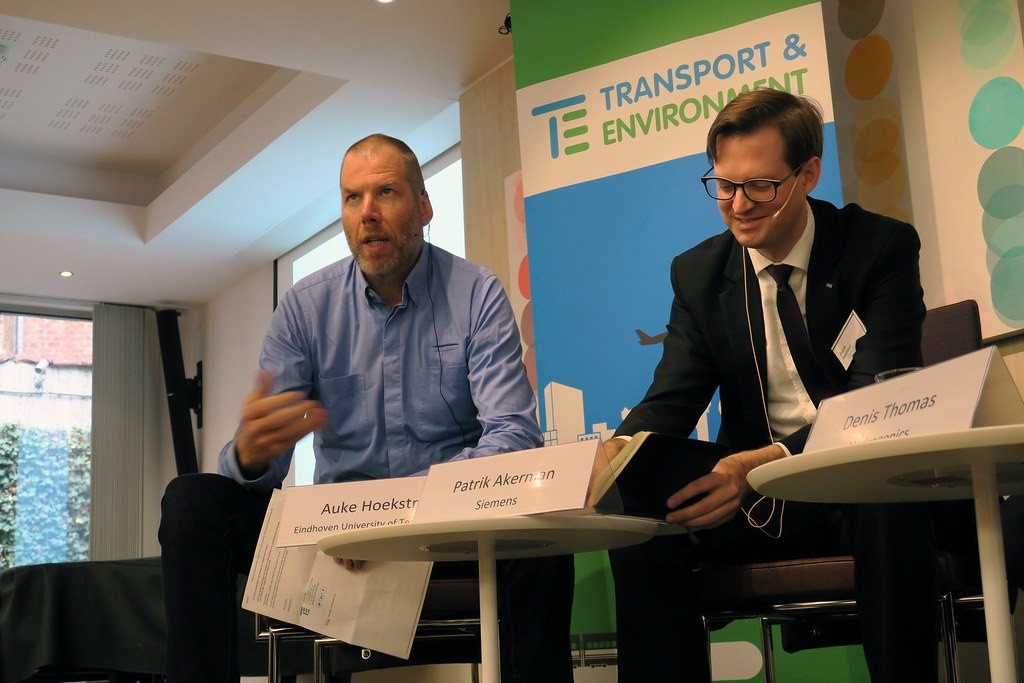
xmin=255 ymin=575 xmax=500 ymax=683
xmin=745 ymin=298 xmax=1002 ymax=683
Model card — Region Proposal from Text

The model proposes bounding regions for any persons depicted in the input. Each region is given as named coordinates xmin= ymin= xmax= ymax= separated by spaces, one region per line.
xmin=594 ymin=86 xmax=940 ymax=682
xmin=157 ymin=133 xmax=573 ymax=683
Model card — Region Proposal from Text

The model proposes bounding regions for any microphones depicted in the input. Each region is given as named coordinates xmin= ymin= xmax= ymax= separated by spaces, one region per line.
xmin=772 ymin=174 xmax=801 ymax=219
xmin=414 ymin=231 xmax=428 ymax=237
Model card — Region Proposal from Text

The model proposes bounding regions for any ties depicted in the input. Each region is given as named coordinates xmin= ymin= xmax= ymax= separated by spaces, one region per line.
xmin=765 ymin=264 xmax=834 ymax=412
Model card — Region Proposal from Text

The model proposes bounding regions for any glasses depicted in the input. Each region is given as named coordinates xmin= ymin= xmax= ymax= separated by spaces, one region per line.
xmin=701 ymin=164 xmax=801 ymax=204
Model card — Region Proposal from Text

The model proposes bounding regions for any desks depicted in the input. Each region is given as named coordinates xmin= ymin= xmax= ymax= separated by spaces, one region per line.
xmin=316 ymin=515 xmax=660 ymax=683
xmin=746 ymin=424 xmax=1024 ymax=683
